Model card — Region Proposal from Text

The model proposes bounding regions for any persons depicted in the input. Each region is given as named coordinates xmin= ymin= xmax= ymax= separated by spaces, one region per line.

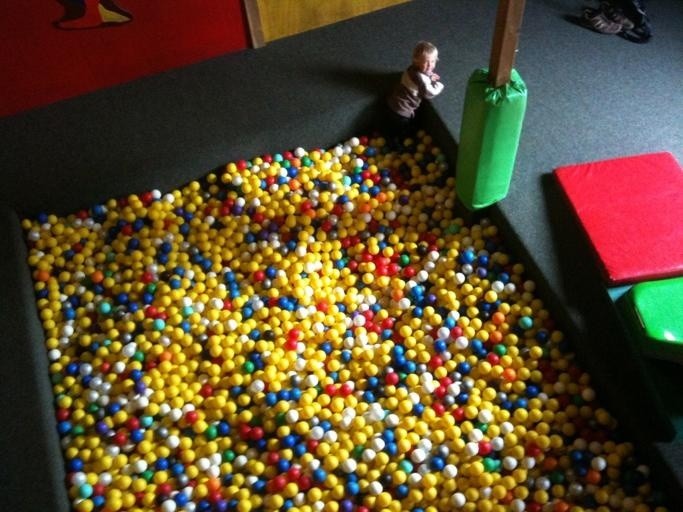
xmin=375 ymin=39 xmax=444 ymax=140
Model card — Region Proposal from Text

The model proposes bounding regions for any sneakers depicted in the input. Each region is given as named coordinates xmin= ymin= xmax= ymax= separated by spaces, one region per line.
xmin=597 ymin=0 xmax=635 ymax=31
xmin=582 ymin=8 xmax=621 ymax=34
